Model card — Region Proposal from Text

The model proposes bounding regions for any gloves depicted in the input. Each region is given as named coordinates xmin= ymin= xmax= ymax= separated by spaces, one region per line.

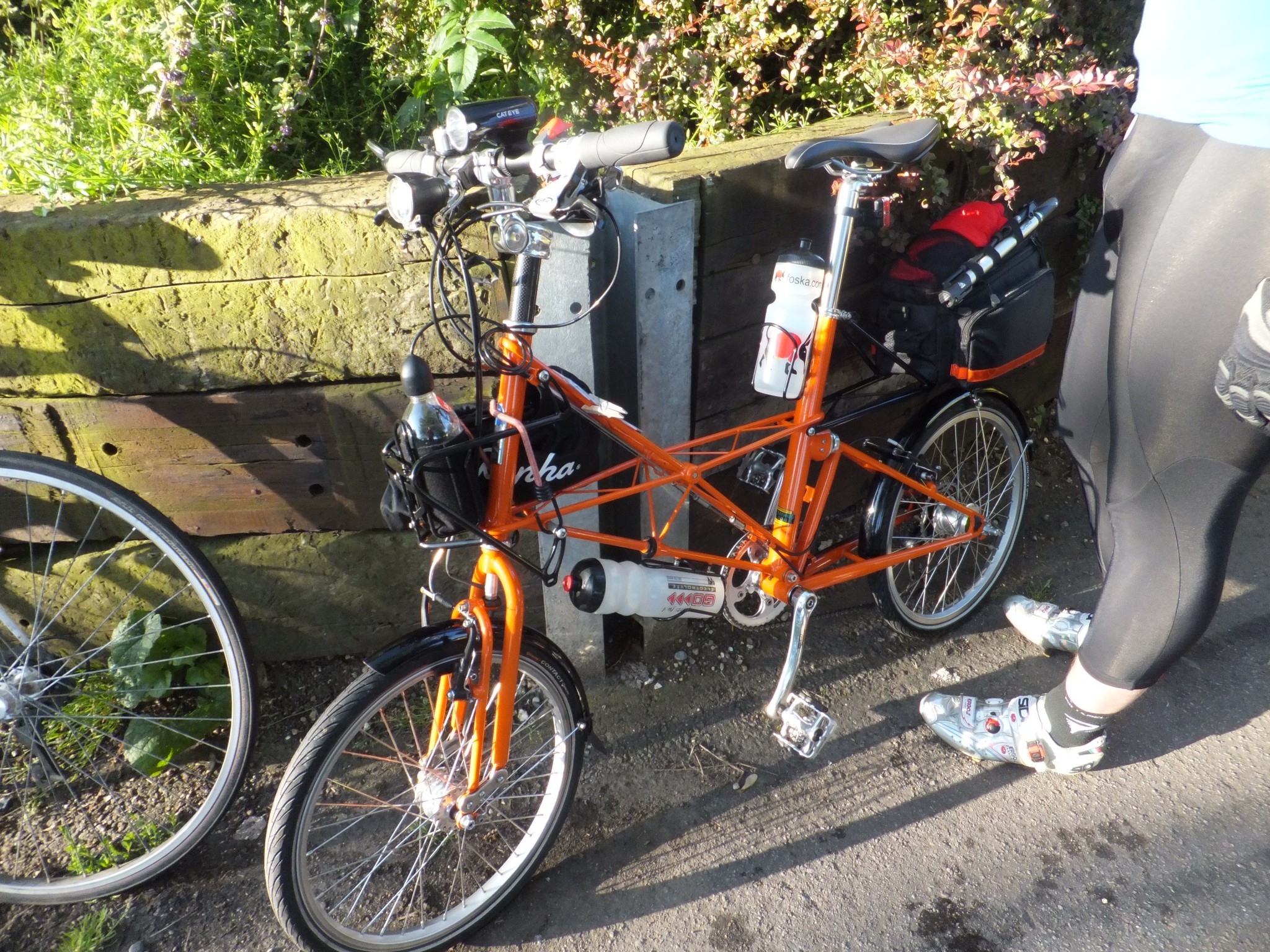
xmin=1214 ymin=278 xmax=1270 ymax=428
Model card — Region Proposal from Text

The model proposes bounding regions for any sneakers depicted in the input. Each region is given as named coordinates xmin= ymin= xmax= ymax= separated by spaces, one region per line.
xmin=919 ymin=692 xmax=1107 ymax=775
xmin=1002 ymin=596 xmax=1094 ymax=654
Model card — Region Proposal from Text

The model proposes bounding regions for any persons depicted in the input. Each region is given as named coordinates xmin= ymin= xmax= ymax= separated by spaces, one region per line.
xmin=918 ymin=0 xmax=1270 ymax=777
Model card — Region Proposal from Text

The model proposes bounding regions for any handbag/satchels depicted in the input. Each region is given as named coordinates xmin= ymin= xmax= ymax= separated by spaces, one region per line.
xmin=866 ymin=233 xmax=1055 ymax=391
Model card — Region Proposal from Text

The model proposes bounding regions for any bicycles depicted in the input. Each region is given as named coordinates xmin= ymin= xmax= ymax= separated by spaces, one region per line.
xmin=0 ymin=449 xmax=262 ymax=910
xmin=263 ymin=95 xmax=1062 ymax=952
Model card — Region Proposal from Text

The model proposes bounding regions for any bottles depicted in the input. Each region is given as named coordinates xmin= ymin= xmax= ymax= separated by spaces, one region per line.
xmin=397 ymin=353 xmax=463 ymax=441
xmin=749 ymin=238 xmax=828 ymax=400
xmin=561 ymin=558 xmax=733 ymax=619
